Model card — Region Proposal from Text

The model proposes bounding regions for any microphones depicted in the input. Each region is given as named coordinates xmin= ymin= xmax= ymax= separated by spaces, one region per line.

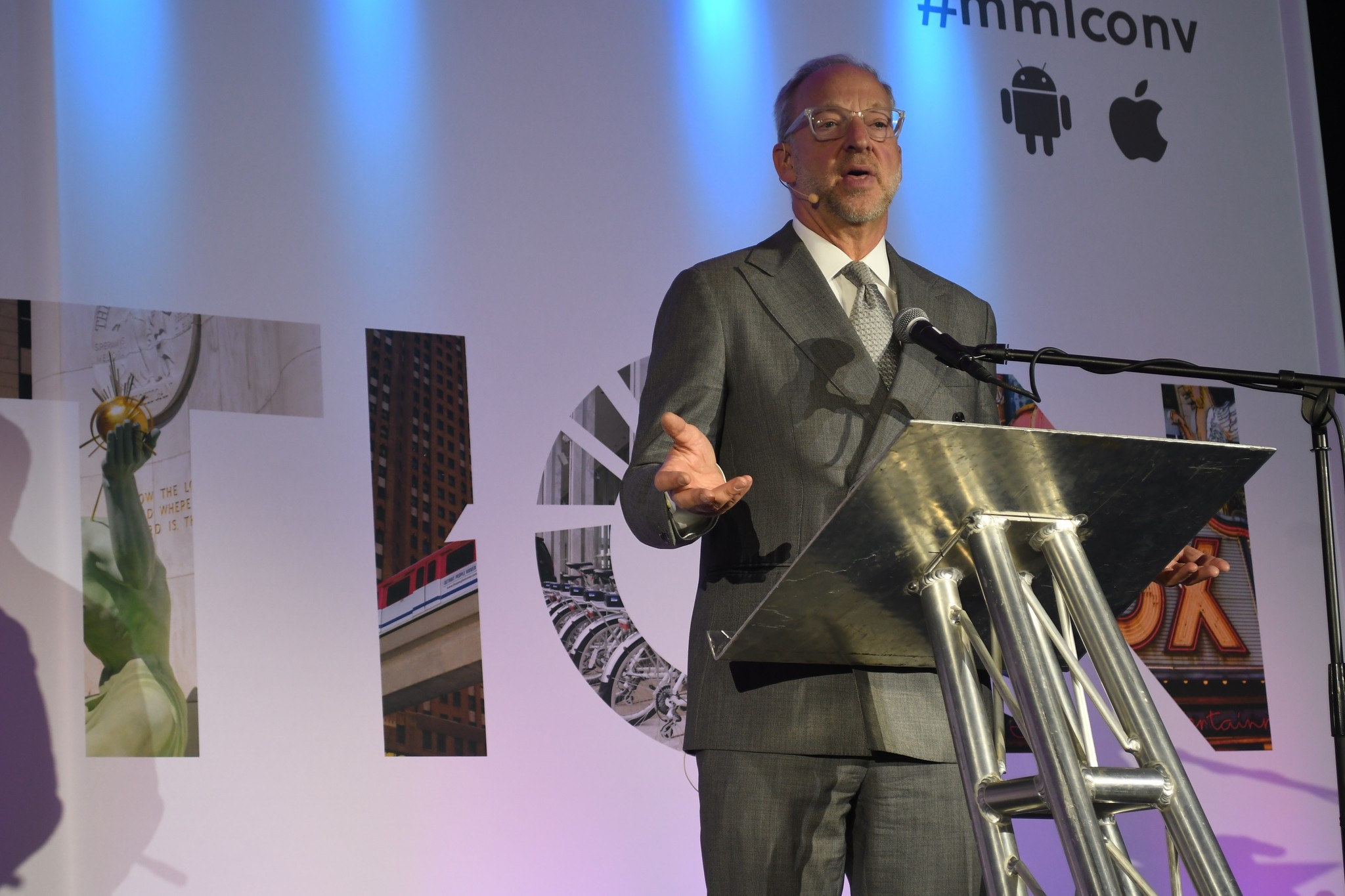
xmin=787 ymin=183 xmax=819 ymax=203
xmin=891 ymin=307 xmax=993 ymax=384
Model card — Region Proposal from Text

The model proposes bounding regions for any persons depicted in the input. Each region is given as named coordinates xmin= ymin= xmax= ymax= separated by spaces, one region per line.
xmin=619 ymin=49 xmax=999 ymax=895
xmin=82 ymin=419 xmax=190 ymax=755
xmin=1171 ymin=384 xmax=1239 ymax=442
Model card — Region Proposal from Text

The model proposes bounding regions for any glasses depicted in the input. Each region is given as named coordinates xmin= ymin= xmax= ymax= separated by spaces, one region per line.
xmin=783 ymin=108 xmax=905 ymax=141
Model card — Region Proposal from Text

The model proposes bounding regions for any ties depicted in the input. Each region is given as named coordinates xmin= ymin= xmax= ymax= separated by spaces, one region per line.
xmin=842 ymin=260 xmax=903 ymax=390
